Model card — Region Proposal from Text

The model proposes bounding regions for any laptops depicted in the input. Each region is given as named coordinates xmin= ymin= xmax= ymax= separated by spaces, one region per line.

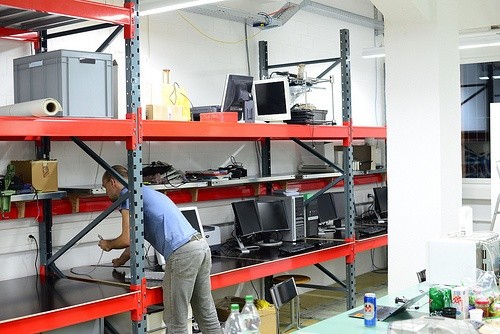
xmin=348 ymin=291 xmax=429 ymax=322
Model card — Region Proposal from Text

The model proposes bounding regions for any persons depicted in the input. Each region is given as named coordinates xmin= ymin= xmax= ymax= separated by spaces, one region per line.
xmin=98 ymin=165 xmax=223 ymax=334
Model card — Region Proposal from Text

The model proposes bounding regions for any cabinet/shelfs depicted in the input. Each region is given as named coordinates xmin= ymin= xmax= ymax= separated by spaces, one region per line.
xmin=0 ymin=0 xmax=388 ymax=334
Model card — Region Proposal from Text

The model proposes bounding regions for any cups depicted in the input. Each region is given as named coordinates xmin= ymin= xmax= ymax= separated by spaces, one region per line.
xmin=469 ymin=309 xmax=483 ymax=322
xmin=441 ymin=307 xmax=457 ymax=320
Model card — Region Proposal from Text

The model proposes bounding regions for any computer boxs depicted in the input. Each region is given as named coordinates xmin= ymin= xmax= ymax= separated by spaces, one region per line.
xmin=258 ymin=195 xmax=319 ymax=242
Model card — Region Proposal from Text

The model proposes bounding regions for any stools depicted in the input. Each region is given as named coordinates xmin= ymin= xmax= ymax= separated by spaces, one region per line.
xmin=272 ymin=274 xmax=311 ymax=334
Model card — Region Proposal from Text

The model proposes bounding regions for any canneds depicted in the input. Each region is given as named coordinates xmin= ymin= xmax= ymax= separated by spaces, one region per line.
xmin=429 ymin=283 xmax=470 ymax=319
xmin=364 ymin=293 xmax=377 ymax=328
xmin=474 ymin=300 xmax=489 ymax=317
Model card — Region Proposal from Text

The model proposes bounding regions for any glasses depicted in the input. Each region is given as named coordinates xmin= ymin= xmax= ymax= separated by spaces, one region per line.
xmin=101 ymin=181 xmax=109 ymax=191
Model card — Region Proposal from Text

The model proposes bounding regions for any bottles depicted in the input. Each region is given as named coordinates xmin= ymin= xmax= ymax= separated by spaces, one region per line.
xmin=240 ymin=295 xmax=261 ymax=334
xmin=155 ymin=68 xmax=175 ymax=120
xmin=364 ymin=293 xmax=376 ymax=327
xmin=223 ymin=304 xmax=246 ymax=334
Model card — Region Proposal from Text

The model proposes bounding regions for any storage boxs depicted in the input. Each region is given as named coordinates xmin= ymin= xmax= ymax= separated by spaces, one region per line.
xmin=13 ymin=48 xmax=114 ymax=118
xmin=214 ymin=296 xmax=276 ymax=334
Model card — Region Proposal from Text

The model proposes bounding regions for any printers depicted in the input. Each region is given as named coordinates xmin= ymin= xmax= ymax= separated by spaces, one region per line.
xmin=201 ymin=225 xmax=220 ymax=256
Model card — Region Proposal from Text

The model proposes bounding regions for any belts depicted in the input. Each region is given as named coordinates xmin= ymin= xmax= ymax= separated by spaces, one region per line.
xmin=190 ymin=234 xmax=202 ymax=241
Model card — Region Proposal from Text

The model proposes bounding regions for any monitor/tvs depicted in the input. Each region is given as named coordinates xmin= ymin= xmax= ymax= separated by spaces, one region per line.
xmin=316 ymin=192 xmax=345 ymax=228
xmin=372 ymin=187 xmax=387 ymax=219
xmin=220 ymin=74 xmax=291 ymax=124
xmin=155 ymin=207 xmax=206 ymax=265
xmin=231 ymin=200 xmax=291 ymax=254
